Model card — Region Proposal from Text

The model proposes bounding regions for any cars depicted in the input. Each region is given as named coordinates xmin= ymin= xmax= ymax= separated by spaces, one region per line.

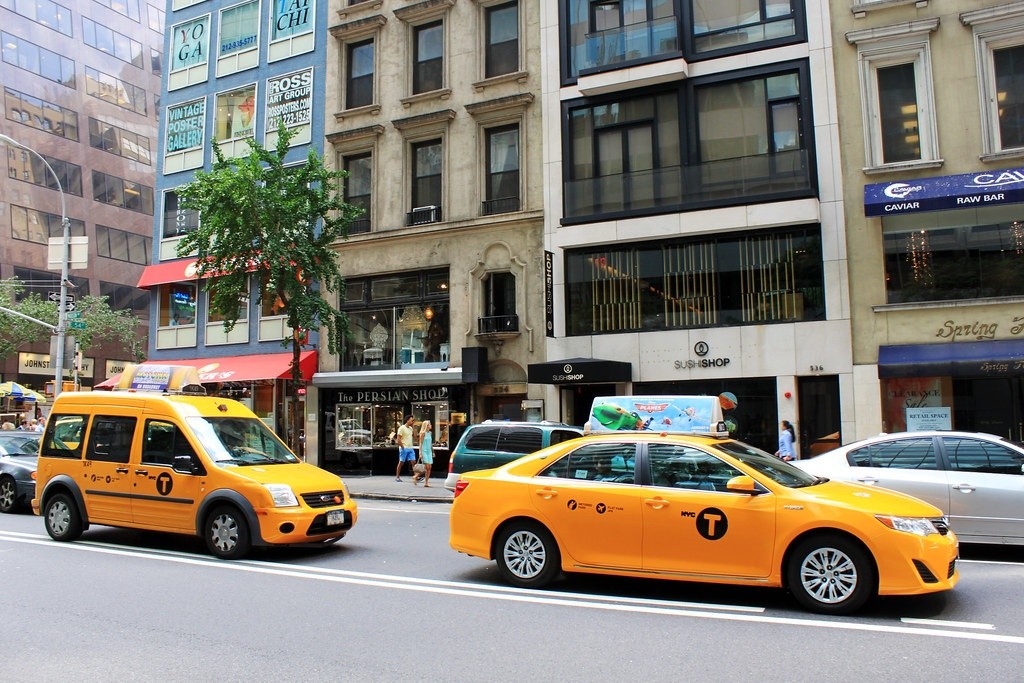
xmin=782 ymin=430 xmax=1024 ymax=547
xmin=0 ymin=431 xmax=72 ymax=512
xmin=447 ymin=392 xmax=964 ymax=617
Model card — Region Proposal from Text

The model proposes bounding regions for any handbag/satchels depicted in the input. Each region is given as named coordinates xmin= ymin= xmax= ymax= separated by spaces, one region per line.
xmin=413 ymin=457 xmax=426 ymax=473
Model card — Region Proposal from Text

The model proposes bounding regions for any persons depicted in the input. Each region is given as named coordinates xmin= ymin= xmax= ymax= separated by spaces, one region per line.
xmin=412 ymin=420 xmax=436 ymax=487
xmin=774 ymin=420 xmax=796 ymax=462
xmin=186 ymin=315 xmax=195 ymax=324
xmin=168 ymin=313 xmax=182 ymax=326
xmin=1 ymin=416 xmax=47 ymax=433
xmin=238 ymin=96 xmax=255 ymax=128
xmin=394 ymin=414 xmax=424 ymax=483
xmin=224 ymin=432 xmax=271 ymax=460
xmin=591 ymin=450 xmax=635 ymax=484
xmin=298 ymin=432 xmax=304 ymax=438
xmin=690 ymin=453 xmax=717 ymax=491
xmin=154 ymin=430 xmax=181 ymax=464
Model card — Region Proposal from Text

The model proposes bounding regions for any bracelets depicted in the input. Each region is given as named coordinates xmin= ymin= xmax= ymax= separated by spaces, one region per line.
xmin=401 ymin=445 xmax=403 ymax=447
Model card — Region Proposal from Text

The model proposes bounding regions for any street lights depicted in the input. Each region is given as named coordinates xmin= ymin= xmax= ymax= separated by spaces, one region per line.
xmin=0 ymin=132 xmax=71 ymax=395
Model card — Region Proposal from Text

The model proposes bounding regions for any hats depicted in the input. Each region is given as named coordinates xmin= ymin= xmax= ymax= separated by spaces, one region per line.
xmin=404 ymin=415 xmax=413 ymax=424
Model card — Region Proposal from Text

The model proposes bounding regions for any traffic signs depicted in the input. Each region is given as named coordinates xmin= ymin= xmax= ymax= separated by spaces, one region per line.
xmin=49 ymin=292 xmax=75 ymax=303
xmin=65 ymin=321 xmax=88 ymax=331
xmin=56 ymin=302 xmax=76 ymax=312
xmin=66 ymin=311 xmax=84 ymax=320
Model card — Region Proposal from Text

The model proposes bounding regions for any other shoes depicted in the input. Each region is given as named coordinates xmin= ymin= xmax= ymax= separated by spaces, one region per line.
xmin=424 ymin=484 xmax=430 ymax=487
xmin=412 ymin=477 xmax=417 ymax=485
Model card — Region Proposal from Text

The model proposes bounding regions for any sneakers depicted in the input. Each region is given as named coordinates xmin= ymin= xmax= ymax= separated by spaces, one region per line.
xmin=417 ymin=478 xmax=423 ymax=482
xmin=395 ymin=478 xmax=402 ymax=482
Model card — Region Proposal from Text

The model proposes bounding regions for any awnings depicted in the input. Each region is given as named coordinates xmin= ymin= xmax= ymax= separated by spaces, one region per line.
xmin=0 ymin=380 xmax=31 ymax=414
xmin=863 ymin=165 xmax=1024 ymax=218
xmin=6 ymin=388 xmax=47 ymax=407
xmin=93 ymin=350 xmax=318 ymax=391
xmin=876 ymin=338 xmax=1024 ymax=380
xmin=527 ymin=357 xmax=632 ymax=384
xmin=135 ymin=241 xmax=321 ymax=291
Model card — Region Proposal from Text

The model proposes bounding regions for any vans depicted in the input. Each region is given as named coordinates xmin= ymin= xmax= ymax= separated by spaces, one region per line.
xmin=31 ymin=388 xmax=359 ymax=559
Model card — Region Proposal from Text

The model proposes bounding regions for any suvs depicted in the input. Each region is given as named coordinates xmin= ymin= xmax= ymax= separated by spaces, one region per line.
xmin=443 ymin=419 xmax=636 ymax=498
xmin=41 ymin=416 xmax=174 ymax=457
